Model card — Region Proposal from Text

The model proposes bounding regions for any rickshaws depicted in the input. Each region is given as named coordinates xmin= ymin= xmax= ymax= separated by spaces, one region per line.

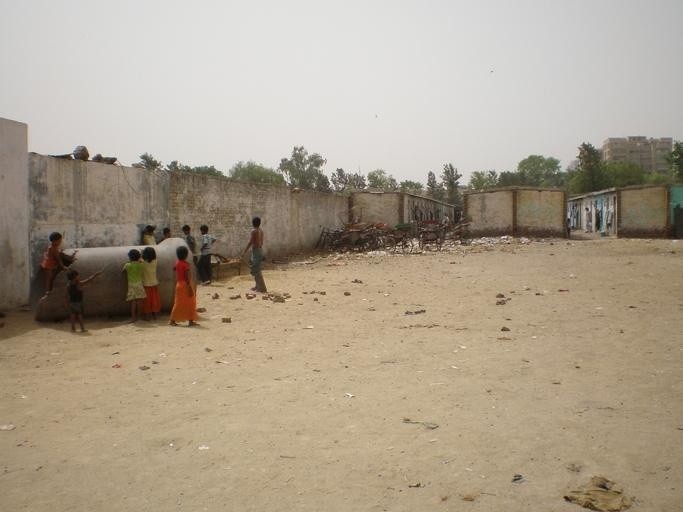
xmin=418 ymin=216 xmax=448 ymax=252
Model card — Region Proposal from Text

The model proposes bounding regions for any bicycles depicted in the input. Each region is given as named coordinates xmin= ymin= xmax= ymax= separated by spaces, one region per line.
xmin=384 ymin=226 xmax=413 ymax=254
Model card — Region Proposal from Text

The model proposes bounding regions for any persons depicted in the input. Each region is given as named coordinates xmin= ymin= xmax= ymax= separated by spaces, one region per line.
xmin=439 ymin=213 xmax=449 ymax=226
xmin=139 ymin=247 xmax=162 ymax=322
xmin=158 ymin=227 xmax=170 ymax=243
xmin=140 ymin=225 xmax=157 ymax=245
xmin=121 ymin=249 xmax=146 ymax=322
xmin=195 ymin=226 xmax=217 ymax=283
xmin=64 ymin=265 xmax=104 ymax=333
xmin=567 ymin=200 xmax=615 ymax=234
xmin=167 ymin=245 xmax=200 ymax=328
xmin=180 ymin=224 xmax=195 ymax=264
xmin=39 ymin=230 xmax=77 ymax=294
xmin=241 ymin=217 xmax=266 ymax=293
